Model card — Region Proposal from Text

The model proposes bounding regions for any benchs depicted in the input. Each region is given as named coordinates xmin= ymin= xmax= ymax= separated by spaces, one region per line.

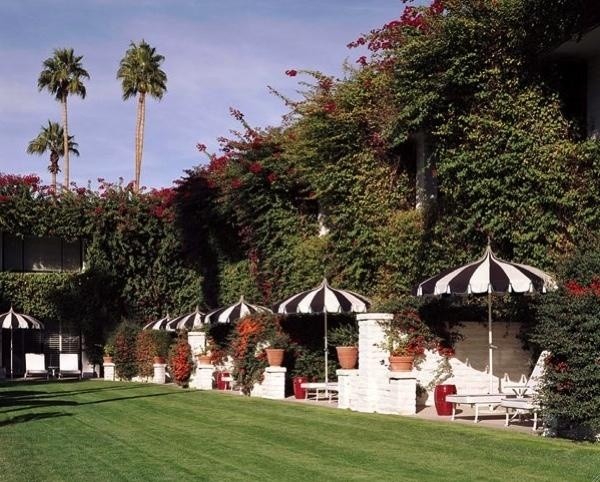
xmin=300 ymin=382 xmax=338 ymax=405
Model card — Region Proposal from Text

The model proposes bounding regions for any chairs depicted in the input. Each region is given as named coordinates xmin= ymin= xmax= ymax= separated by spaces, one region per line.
xmin=24 ymin=353 xmax=82 ymax=382
xmin=445 ymin=351 xmax=550 ymax=431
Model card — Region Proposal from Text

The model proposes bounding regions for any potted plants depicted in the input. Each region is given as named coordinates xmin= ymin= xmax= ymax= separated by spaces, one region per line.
xmin=153 ymin=349 xmax=164 ymax=364
xmin=389 ymin=347 xmax=415 ymax=370
xmin=199 ymin=345 xmax=211 ymax=364
xmin=328 ymin=323 xmax=358 ymax=369
xmin=265 ymin=335 xmax=288 ymax=366
xmin=103 ymin=344 xmax=115 ymax=362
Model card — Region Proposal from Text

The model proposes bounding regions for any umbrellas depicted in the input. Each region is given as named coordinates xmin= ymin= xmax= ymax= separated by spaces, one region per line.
xmin=143 ymin=294 xmax=273 ymax=332
xmin=411 ymin=234 xmax=558 ymax=392
xmin=272 ymin=272 xmax=374 ymax=380
xmin=0 ymin=302 xmax=46 ymax=372
